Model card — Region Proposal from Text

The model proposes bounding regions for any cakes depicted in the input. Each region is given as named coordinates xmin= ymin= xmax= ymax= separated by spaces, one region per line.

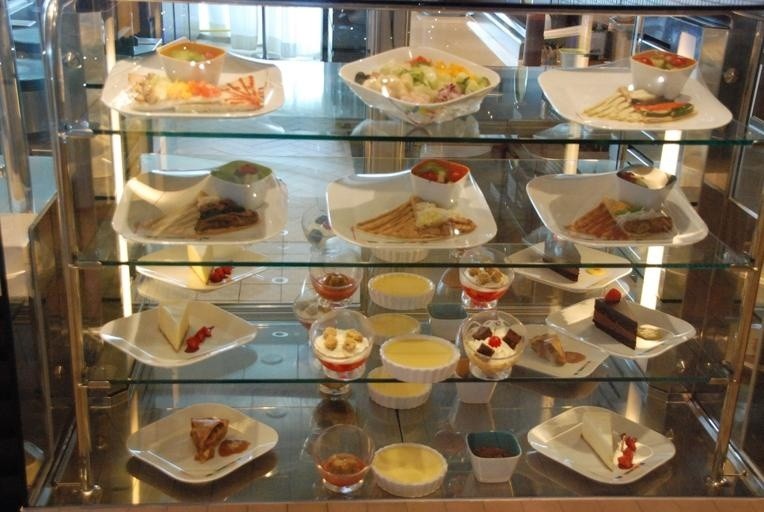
xmin=316 ymin=327 xmax=368 ymax=377
xmin=318 ymin=272 xmax=355 ymax=300
xmin=577 ymin=408 xmax=636 ymax=469
xmin=293 ymin=289 xmax=332 ymax=327
xmin=177 ymin=74 xmax=265 ymax=112
xmin=566 ymin=196 xmax=678 ymax=241
xmin=123 ymin=68 xmax=226 ymax=111
xmin=532 ymin=332 xmax=585 ymax=367
xmin=158 ymin=299 xmax=216 ymax=355
xmin=182 ymin=244 xmax=235 ymax=282
xmin=593 ymin=290 xmax=669 ymax=349
xmin=580 ymin=87 xmax=698 ymax=122
xmin=468 ymin=319 xmax=521 ymax=373
xmin=462 ymin=257 xmax=510 ymax=304
xmin=135 ymin=191 xmax=260 ymax=239
xmin=362 ymin=197 xmax=475 ymax=241
xmin=187 ymin=415 xmax=249 ymax=461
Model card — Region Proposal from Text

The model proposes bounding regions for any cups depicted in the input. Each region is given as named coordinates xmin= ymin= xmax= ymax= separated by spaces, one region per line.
xmin=615 ymin=163 xmax=677 ymax=210
xmin=428 ymin=300 xmax=469 ymax=341
xmin=461 ymin=311 xmax=527 ymax=382
xmin=326 ymin=168 xmax=498 ymax=250
xmin=465 ymin=431 xmax=522 ymax=484
xmin=302 ymin=204 xmax=336 ymax=248
xmin=451 ymin=405 xmax=496 ymax=435
xmin=309 ymin=308 xmax=377 ymax=380
xmin=308 ymin=249 xmax=365 ymax=309
xmin=313 ymin=424 xmax=376 ymax=493
xmin=157 ymin=41 xmax=226 ymax=87
xmin=411 ymin=158 xmax=470 ymax=209
xmin=459 ymin=247 xmax=515 ymax=312
xmin=630 ymin=50 xmax=697 ymax=100
xmin=454 ymin=359 xmax=498 ymax=404
xmin=294 ymin=286 xmax=347 ymax=330
xmin=210 ymin=160 xmax=273 ymax=209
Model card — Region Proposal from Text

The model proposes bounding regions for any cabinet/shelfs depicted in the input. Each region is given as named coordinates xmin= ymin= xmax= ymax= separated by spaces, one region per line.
xmin=40 ymin=1 xmax=763 ymax=512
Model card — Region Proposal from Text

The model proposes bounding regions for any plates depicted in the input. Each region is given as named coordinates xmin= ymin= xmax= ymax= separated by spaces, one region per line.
xmin=537 ymin=56 xmax=733 ymax=132
xmin=338 ymin=46 xmax=501 ymax=125
xmin=544 ymin=297 xmax=696 ymax=360
xmin=514 ymin=324 xmax=610 ymax=378
xmin=111 ymin=173 xmax=289 ymax=245
xmin=126 ymin=402 xmax=279 ymax=485
xmin=99 ymin=301 xmax=259 ymax=368
xmin=135 ymin=246 xmax=271 ymax=293
xmin=366 ymin=364 xmax=434 ymax=411
xmin=525 ymin=170 xmax=710 ymax=248
xmin=371 ymin=442 xmax=448 ymax=498
xmin=366 ymin=313 xmax=421 ymax=347
xmin=526 ymin=405 xmax=677 ymax=486
xmin=367 ymin=271 xmax=434 ymax=311
xmin=100 ymin=54 xmax=285 ymax=120
xmin=504 ymin=240 xmax=633 ymax=294
xmin=381 ymin=334 xmax=462 ymax=384
